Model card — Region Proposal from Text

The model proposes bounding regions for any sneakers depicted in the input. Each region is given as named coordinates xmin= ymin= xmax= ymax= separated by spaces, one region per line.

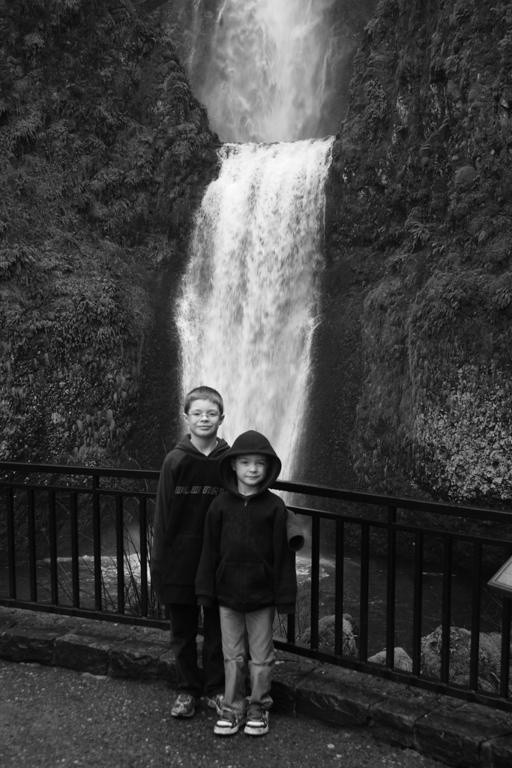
xmin=208 ymin=695 xmax=225 ymax=715
xmin=213 ymin=712 xmax=245 ymax=735
xmin=243 ymin=710 xmax=268 ymax=735
xmin=171 ymin=693 xmax=196 ymax=717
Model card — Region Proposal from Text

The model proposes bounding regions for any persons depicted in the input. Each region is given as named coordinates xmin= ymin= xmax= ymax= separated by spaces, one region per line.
xmin=194 ymin=429 xmax=296 ymax=739
xmin=147 ymin=383 xmax=231 ymax=720
